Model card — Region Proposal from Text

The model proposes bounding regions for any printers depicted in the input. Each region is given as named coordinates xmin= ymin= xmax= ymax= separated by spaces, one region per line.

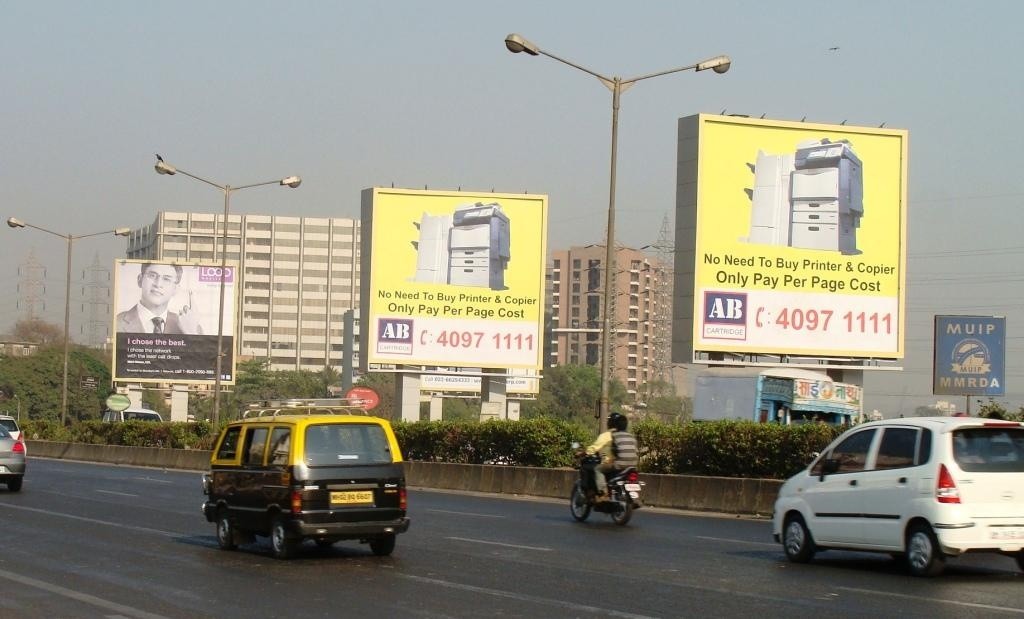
xmin=785 ymin=143 xmax=862 ymax=254
xmin=446 ymin=205 xmax=511 ymax=289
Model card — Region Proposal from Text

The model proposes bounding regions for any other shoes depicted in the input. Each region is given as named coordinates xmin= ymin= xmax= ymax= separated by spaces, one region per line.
xmin=594 ymin=495 xmax=610 ymax=503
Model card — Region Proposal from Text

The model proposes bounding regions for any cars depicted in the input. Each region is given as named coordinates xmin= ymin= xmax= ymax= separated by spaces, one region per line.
xmin=0 ymin=414 xmax=28 ymax=493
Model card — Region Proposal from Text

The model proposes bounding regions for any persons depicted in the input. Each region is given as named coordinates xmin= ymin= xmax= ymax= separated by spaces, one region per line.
xmin=574 ymin=412 xmax=638 ymax=503
xmin=116 ymin=263 xmax=184 ymax=334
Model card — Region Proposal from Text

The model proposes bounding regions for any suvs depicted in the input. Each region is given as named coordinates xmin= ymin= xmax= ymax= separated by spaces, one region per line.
xmin=102 ymin=408 xmax=164 ymax=424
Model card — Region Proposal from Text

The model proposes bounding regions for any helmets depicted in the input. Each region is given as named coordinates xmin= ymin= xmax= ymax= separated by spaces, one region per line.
xmin=607 ymin=412 xmax=620 ymax=428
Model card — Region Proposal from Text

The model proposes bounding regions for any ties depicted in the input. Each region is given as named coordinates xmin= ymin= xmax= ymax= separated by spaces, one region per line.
xmin=152 ymin=317 xmax=164 ymax=333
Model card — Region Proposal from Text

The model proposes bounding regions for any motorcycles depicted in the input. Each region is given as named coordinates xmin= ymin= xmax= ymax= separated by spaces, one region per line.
xmin=568 ymin=442 xmax=647 ymax=526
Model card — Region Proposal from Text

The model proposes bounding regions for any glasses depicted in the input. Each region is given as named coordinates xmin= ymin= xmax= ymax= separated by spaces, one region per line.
xmin=143 ymin=271 xmax=175 ymax=288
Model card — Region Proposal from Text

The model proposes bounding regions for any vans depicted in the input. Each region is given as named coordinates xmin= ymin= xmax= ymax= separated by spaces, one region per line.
xmin=772 ymin=412 xmax=1024 ymax=581
xmin=201 ymin=397 xmax=412 ymax=560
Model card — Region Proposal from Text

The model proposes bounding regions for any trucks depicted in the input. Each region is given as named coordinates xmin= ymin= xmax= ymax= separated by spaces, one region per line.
xmin=692 ymin=363 xmax=865 ymax=429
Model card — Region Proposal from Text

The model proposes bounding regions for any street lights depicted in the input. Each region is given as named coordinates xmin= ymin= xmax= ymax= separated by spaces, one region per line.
xmin=7 ymin=216 xmax=130 ymax=426
xmin=154 ymin=159 xmax=303 ymax=440
xmin=501 ymin=31 xmax=731 ymax=460
